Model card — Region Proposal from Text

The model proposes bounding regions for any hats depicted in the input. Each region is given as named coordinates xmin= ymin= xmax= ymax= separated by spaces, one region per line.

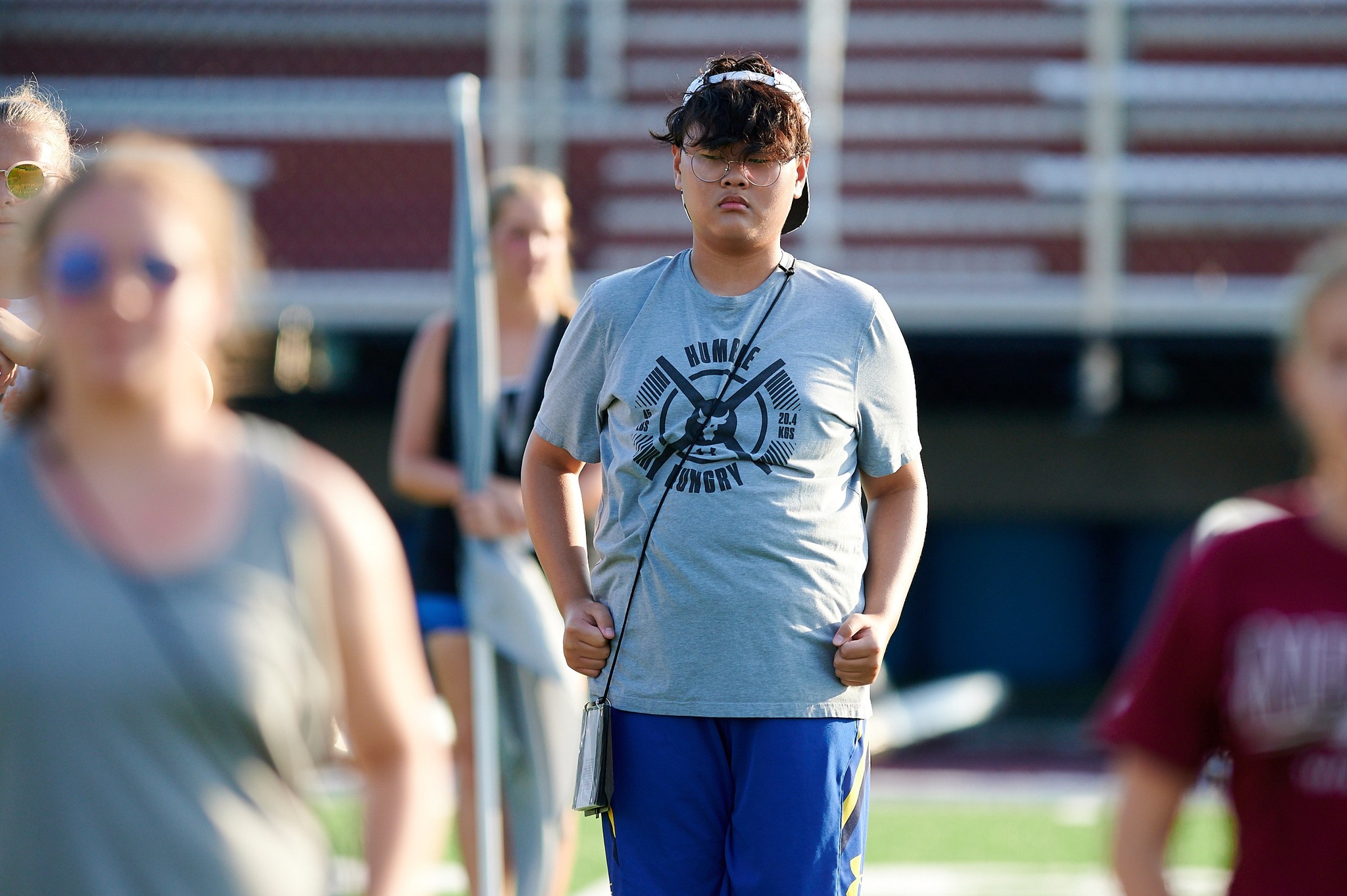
xmin=681 ymin=59 xmax=810 ymax=235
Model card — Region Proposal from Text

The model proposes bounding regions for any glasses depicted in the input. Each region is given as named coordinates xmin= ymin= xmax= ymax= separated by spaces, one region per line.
xmin=0 ymin=161 xmax=74 ymax=204
xmin=58 ymin=254 xmax=181 ymax=298
xmin=680 ymin=142 xmax=805 ymax=188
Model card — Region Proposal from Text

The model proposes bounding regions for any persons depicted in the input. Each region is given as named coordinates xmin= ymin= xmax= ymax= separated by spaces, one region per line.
xmin=390 ymin=165 xmax=605 ymax=896
xmin=2 ymin=134 xmax=458 ymax=893
xmin=517 ymin=48 xmax=929 ymax=896
xmin=1091 ymin=237 xmax=1347 ymax=895
xmin=1 ymin=93 xmax=75 ymax=412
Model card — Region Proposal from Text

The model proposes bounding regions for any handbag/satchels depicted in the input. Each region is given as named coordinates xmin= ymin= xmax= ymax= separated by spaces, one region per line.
xmin=459 ymin=544 xmax=573 ymax=685
xmin=569 ymin=698 xmax=615 ymax=820
